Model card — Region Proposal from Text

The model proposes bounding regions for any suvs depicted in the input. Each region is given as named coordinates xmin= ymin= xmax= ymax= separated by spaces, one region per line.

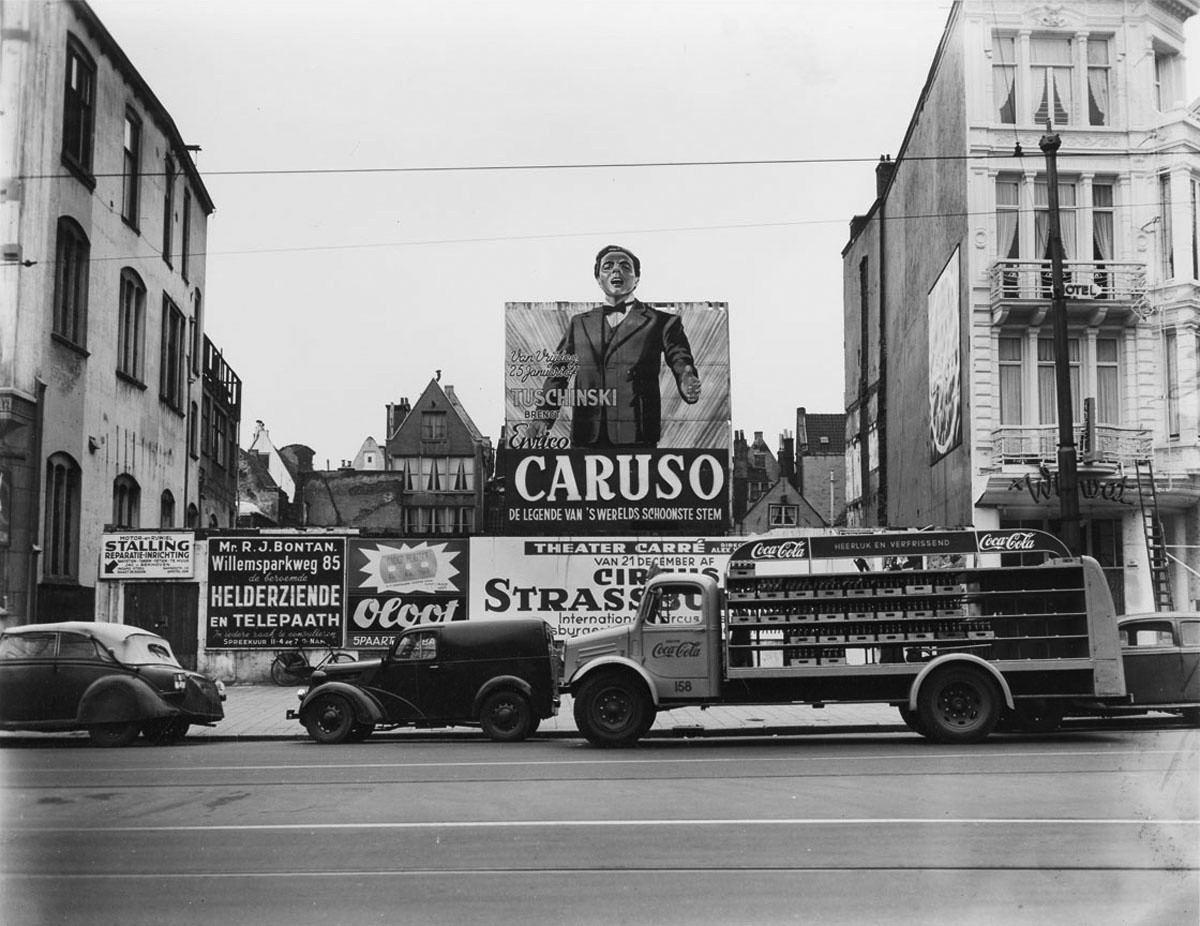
xmin=285 ymin=615 xmax=563 ymax=745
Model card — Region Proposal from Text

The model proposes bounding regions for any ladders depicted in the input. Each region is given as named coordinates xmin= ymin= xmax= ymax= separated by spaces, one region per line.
xmin=1135 ymin=458 xmax=1174 ymax=644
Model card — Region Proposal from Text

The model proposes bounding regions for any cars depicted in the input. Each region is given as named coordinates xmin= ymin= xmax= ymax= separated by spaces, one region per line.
xmin=1116 ymin=611 xmax=1200 ymax=730
xmin=0 ymin=621 xmax=227 ymax=749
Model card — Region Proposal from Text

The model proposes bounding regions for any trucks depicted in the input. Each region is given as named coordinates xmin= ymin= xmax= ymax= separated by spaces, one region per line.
xmin=563 ymin=525 xmax=1136 ymax=752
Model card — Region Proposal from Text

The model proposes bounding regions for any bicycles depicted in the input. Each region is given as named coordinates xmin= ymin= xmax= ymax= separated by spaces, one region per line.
xmin=270 ymin=648 xmax=357 ymax=688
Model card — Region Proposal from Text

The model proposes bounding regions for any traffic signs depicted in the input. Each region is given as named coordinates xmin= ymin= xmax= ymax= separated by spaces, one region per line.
xmin=98 ymin=530 xmax=195 ymax=580
